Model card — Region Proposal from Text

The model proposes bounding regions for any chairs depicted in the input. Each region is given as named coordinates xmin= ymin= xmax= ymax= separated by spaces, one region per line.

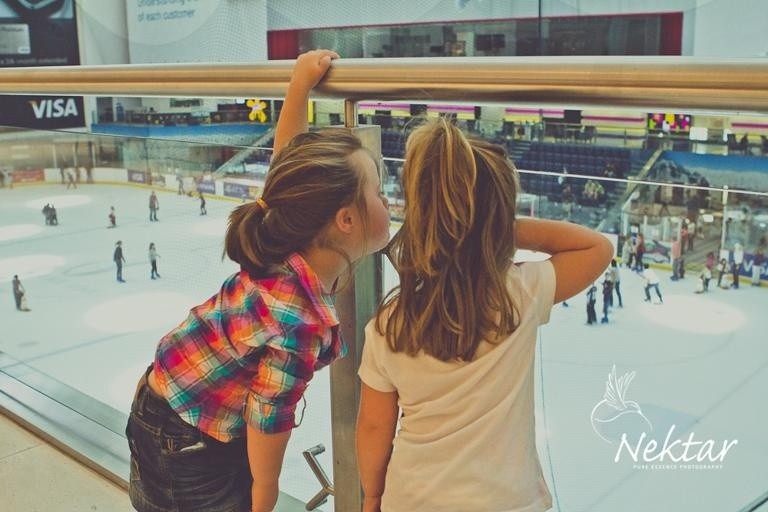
xmin=469 ymin=112 xmax=633 ymax=207
xmin=374 ymin=117 xmax=408 ymax=196
xmin=726 ymin=131 xmax=767 ymax=157
xmin=244 ymin=136 xmax=273 ymax=169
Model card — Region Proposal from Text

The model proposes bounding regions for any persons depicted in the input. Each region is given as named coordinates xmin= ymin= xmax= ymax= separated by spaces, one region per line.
xmin=12 ymin=273 xmax=25 ymax=311
xmin=40 ymin=100 xmax=768 ymax=326
xmin=355 ymin=109 xmax=613 ymax=512
xmin=124 ymin=49 xmax=392 ymax=512
